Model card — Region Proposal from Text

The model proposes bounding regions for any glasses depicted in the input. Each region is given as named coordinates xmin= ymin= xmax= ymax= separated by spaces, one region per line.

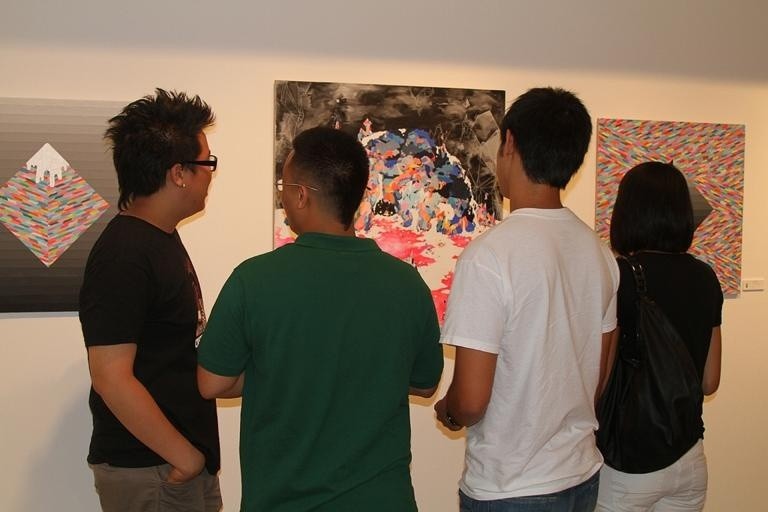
xmin=277 ymin=177 xmax=317 ymax=192
xmin=173 ymin=155 xmax=217 ymax=172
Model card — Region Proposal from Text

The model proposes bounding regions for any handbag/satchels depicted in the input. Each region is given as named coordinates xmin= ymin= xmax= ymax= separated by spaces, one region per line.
xmin=594 ymin=255 xmax=700 ymax=442
